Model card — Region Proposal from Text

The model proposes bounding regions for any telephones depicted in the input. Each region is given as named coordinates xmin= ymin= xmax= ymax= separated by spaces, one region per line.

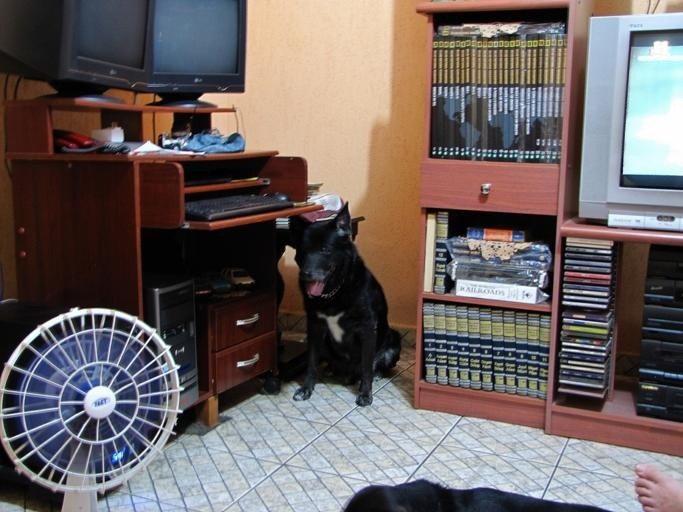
xmin=53 ymin=127 xmax=103 ymax=150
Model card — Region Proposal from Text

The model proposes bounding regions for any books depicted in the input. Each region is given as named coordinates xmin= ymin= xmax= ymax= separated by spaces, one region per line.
xmin=419 ymin=209 xmax=624 ymax=404
xmin=427 ymin=20 xmax=570 ymax=166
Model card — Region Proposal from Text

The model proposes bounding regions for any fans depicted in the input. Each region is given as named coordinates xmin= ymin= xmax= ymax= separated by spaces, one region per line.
xmin=0 ymin=304 xmax=188 ymax=512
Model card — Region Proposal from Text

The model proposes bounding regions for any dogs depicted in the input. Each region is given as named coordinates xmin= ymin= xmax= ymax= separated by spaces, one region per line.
xmin=342 ymin=478 xmax=614 ymax=512
xmin=287 ymin=201 xmax=402 ymax=407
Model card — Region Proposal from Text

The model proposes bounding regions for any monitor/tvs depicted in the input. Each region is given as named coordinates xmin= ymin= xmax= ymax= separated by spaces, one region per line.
xmin=0 ymin=0 xmax=156 ymax=102
xmin=146 ymin=0 xmax=247 ymax=107
xmin=578 ymin=11 xmax=683 ymax=234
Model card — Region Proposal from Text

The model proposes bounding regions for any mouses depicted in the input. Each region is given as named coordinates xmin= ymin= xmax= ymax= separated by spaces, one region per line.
xmin=269 ymin=189 xmax=288 ymax=201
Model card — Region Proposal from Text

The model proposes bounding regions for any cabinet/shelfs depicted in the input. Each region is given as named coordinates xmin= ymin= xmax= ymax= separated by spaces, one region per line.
xmin=543 ymin=214 xmax=683 ymax=459
xmin=412 ymin=1 xmax=635 ymax=438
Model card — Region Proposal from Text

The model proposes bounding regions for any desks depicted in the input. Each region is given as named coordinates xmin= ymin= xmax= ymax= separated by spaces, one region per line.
xmin=0 ymin=87 xmax=320 ymax=434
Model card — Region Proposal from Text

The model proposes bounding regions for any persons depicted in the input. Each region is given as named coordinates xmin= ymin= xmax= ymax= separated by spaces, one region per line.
xmin=630 ymin=460 xmax=683 ymax=512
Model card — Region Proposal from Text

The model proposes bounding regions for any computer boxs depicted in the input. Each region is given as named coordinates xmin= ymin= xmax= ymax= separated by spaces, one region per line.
xmin=145 ymin=277 xmax=200 ymax=407
xmin=635 ymin=243 xmax=682 ymax=423
xmin=2 ymin=297 xmax=138 ymax=500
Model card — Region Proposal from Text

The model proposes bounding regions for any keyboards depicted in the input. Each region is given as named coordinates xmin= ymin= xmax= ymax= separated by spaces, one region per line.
xmin=184 ymin=194 xmax=295 ymax=221
